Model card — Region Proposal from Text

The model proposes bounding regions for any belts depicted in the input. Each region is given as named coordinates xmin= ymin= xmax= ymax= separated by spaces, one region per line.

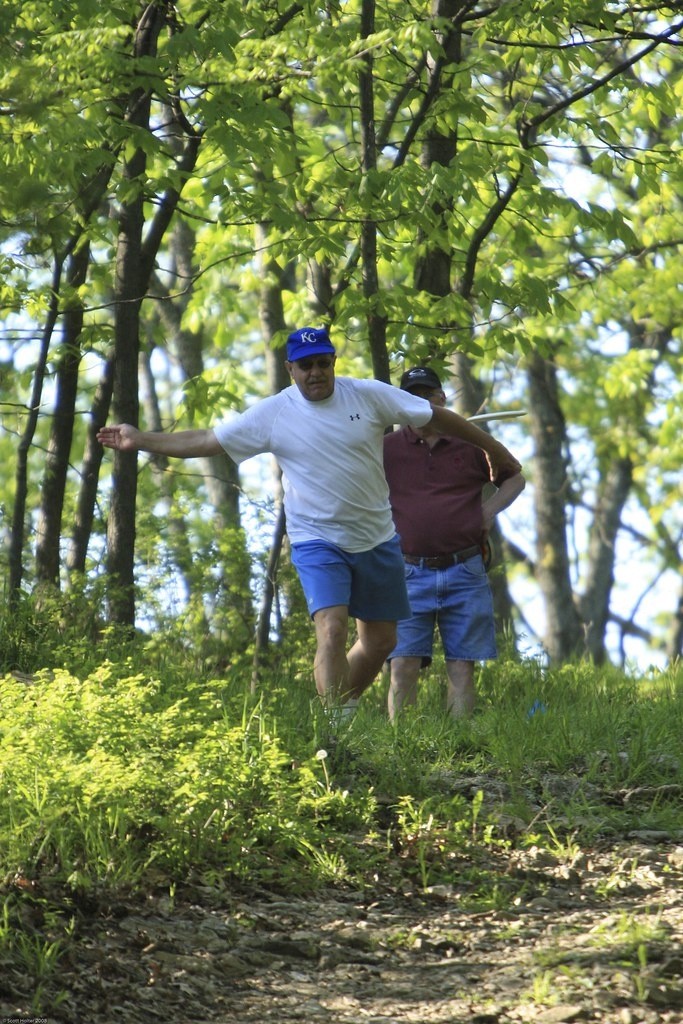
xmin=403 ymin=545 xmax=480 ymax=569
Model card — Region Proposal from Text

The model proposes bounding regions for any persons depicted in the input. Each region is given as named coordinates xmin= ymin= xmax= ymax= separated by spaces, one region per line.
xmin=384 ymin=368 xmax=526 ymax=728
xmin=95 ymin=327 xmax=523 ymax=728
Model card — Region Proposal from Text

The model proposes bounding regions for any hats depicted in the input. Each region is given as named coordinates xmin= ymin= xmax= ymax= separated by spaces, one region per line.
xmin=286 ymin=327 xmax=334 ymax=361
xmin=400 ymin=367 xmax=441 ymax=390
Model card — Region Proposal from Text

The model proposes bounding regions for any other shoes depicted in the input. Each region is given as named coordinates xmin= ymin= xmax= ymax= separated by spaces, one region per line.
xmin=318 ymin=741 xmax=350 ymax=776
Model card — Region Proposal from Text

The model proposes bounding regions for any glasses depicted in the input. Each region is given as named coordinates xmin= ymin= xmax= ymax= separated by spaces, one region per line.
xmin=296 ymin=359 xmax=333 ymax=370
